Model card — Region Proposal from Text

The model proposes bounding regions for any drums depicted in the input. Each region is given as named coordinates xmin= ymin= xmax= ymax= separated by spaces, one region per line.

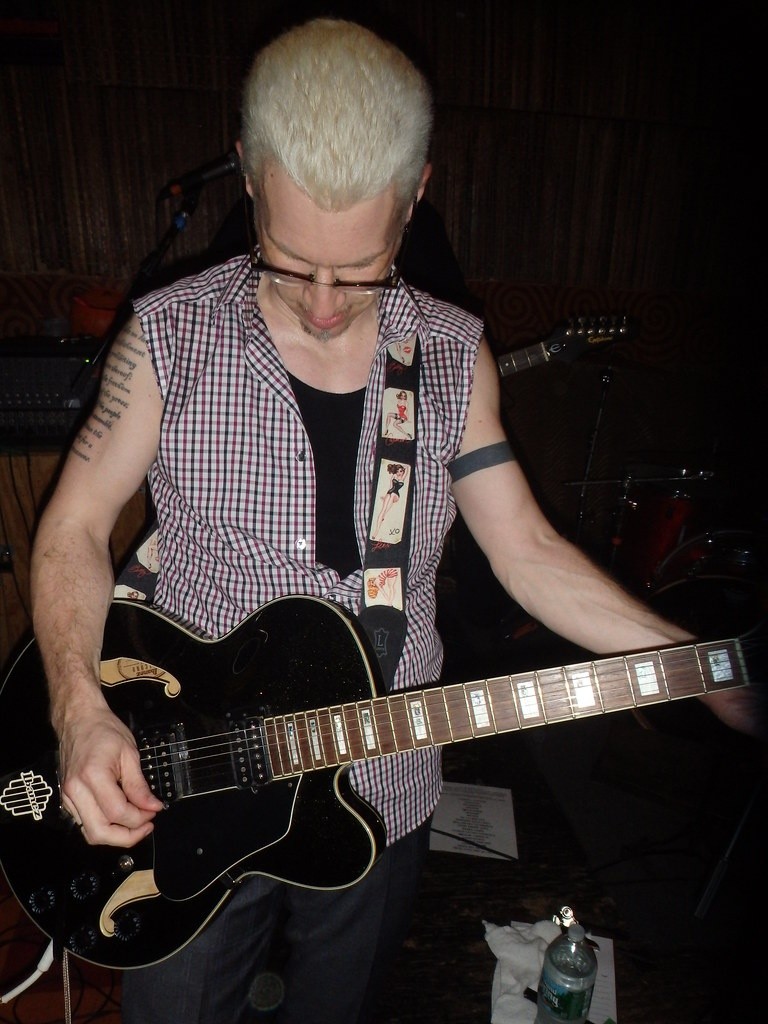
xmin=621 ymin=529 xmax=768 ymax=729
xmin=607 ymin=484 xmax=706 ymax=587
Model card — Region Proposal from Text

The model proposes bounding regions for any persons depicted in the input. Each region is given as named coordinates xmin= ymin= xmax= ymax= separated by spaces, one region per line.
xmin=29 ymin=19 xmax=767 ymax=1024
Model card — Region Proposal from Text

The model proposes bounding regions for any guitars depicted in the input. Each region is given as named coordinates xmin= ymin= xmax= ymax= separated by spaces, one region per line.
xmin=0 ymin=593 xmax=768 ymax=970
xmin=496 ymin=306 xmax=641 ymax=378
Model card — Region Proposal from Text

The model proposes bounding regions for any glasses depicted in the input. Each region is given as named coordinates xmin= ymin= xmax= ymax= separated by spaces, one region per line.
xmin=249 ymin=174 xmax=417 ymax=296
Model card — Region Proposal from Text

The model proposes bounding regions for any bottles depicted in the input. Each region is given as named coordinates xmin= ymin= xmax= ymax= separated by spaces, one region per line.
xmin=534 ymin=925 xmax=598 ymax=1024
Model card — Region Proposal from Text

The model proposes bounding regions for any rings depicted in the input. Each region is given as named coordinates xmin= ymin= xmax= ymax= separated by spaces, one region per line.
xmin=78 ymin=822 xmax=84 ymax=831
